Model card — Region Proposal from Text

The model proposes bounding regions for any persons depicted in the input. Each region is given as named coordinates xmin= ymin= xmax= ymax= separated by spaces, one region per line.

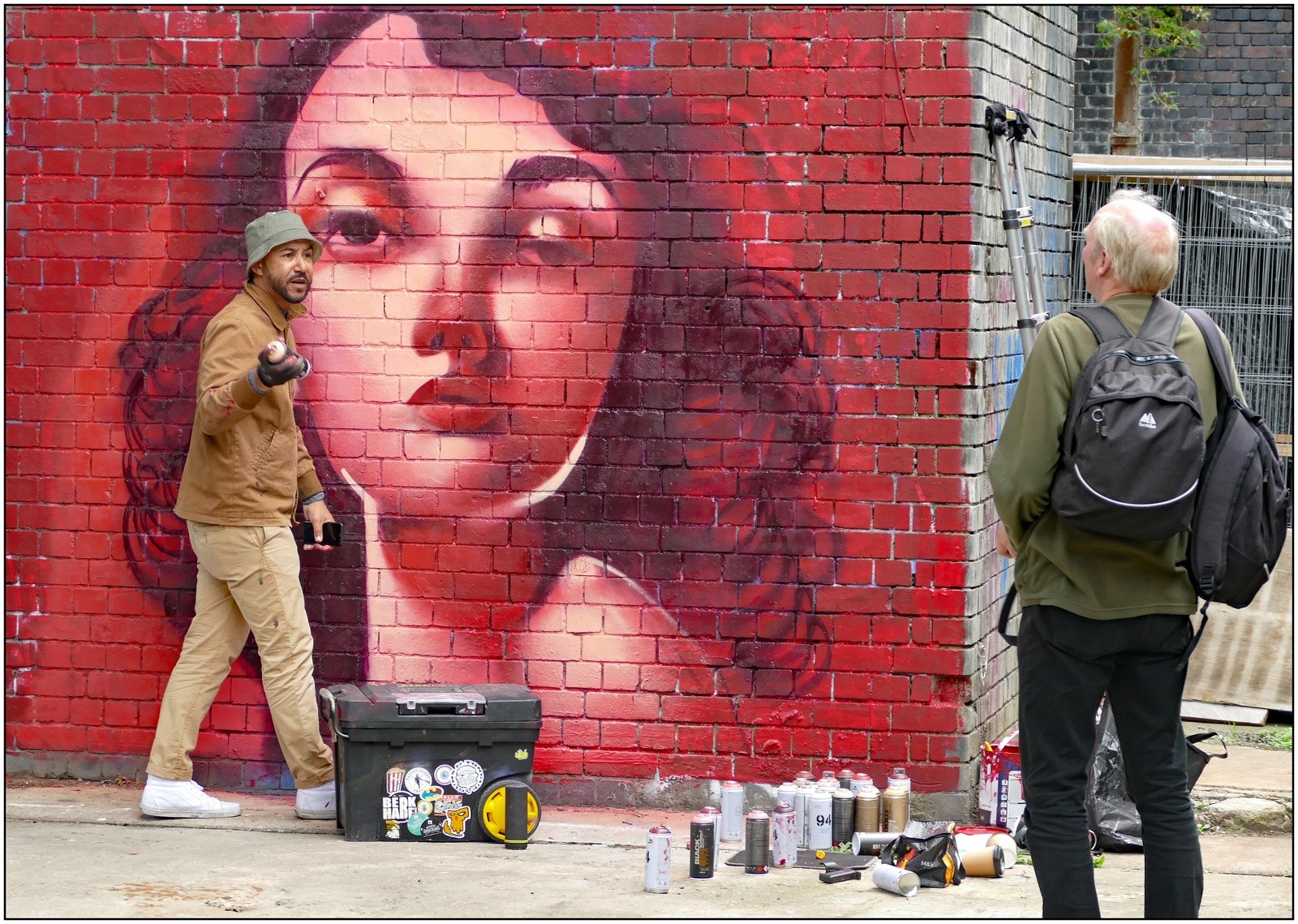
xmin=988 ymin=189 xmax=1245 ymax=921
xmin=140 ymin=209 xmax=340 ymax=818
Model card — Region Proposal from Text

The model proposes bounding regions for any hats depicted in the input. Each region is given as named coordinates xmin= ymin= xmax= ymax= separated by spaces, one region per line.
xmin=245 ymin=210 xmax=322 ymax=279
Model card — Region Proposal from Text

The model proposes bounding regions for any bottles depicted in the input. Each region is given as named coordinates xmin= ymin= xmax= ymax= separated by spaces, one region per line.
xmin=745 ymin=809 xmax=770 ymax=875
xmin=644 ymin=823 xmax=670 ymax=894
xmin=266 ymin=340 xmax=311 ymax=380
xmin=776 ymin=767 xmax=911 ymax=857
xmin=690 ymin=805 xmax=722 ymax=879
xmin=505 ymin=784 xmax=528 ymax=850
xmin=772 ymin=801 xmax=797 ymax=869
xmin=872 ymin=864 xmax=920 ymax=897
xmin=720 ymin=780 xmax=744 ymax=843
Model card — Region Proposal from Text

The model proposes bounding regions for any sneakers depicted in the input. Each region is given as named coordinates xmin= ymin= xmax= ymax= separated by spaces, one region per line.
xmin=295 ymin=780 xmax=338 ymax=818
xmin=139 ymin=772 xmax=243 ymax=818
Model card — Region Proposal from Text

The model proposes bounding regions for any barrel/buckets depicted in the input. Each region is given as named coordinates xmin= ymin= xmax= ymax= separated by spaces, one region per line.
xmin=979 ymin=729 xmax=1026 ymax=838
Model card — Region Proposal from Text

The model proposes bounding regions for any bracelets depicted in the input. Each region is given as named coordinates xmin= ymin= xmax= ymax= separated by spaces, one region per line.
xmin=257 ymin=364 xmax=273 ymax=388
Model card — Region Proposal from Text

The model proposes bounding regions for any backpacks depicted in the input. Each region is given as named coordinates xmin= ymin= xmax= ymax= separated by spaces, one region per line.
xmin=1048 ymin=295 xmax=1208 ymax=540
xmin=1183 ymin=307 xmax=1291 ymax=611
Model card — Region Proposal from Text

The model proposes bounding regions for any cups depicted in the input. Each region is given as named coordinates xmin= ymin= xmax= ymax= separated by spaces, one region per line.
xmin=986 ymin=832 xmax=1017 ymax=868
xmin=962 ymin=845 xmax=1006 ymax=878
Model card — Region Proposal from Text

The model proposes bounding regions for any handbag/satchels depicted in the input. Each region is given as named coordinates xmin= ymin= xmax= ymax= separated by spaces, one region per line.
xmin=1088 ymin=702 xmax=1228 ymax=855
xmin=881 ymin=832 xmax=966 ymax=888
